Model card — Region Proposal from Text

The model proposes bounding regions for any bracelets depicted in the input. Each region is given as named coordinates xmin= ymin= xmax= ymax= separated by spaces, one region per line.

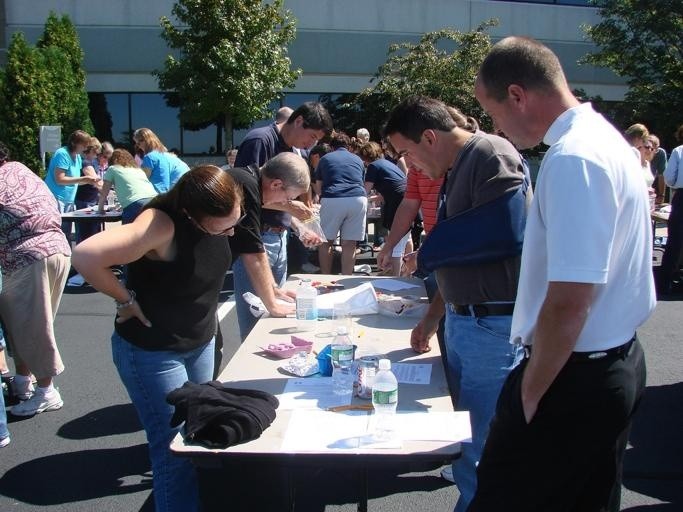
xmin=659 ymin=193 xmax=666 ymax=198
xmin=113 ymin=289 xmax=137 ymax=310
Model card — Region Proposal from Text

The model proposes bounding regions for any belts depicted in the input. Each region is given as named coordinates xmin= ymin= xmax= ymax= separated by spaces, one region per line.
xmin=524 ymin=345 xmax=630 ymax=361
xmin=446 ymin=302 xmax=517 ymax=318
xmin=261 ymin=224 xmax=288 ymax=233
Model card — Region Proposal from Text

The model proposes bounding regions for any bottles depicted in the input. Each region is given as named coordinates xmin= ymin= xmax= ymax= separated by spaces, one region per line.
xmin=294 ymin=278 xmax=319 ymax=336
xmin=372 ymin=359 xmax=398 ymax=442
xmin=331 ymin=327 xmax=354 ymax=397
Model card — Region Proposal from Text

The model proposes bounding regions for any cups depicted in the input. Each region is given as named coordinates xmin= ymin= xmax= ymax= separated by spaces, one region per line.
xmin=330 ymin=303 xmax=353 ymax=335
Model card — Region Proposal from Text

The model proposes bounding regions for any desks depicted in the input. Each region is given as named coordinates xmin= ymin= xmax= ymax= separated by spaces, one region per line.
xmin=167 ymin=273 xmax=464 ymax=512
xmin=62 ymin=204 xmax=123 ymax=232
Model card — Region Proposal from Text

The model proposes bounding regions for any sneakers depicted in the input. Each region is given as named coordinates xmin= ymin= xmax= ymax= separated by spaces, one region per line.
xmin=440 ymin=466 xmax=459 ymax=484
xmin=1 ymin=434 xmax=10 ymax=448
xmin=302 ymin=262 xmax=319 ymax=273
xmin=12 ymin=389 xmax=64 ymax=415
xmin=1 ymin=378 xmax=33 ymax=401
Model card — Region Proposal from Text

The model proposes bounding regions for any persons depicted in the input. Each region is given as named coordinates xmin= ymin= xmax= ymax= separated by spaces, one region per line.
xmin=384 ymin=94 xmax=536 ymax=511
xmin=71 ymin=164 xmax=249 ymax=512
xmin=651 ymin=134 xmax=667 ymax=205
xmin=133 ymin=128 xmax=169 ymax=196
xmin=381 ymin=134 xmax=425 ymax=250
xmin=465 ymin=36 xmax=659 ymax=510
xmin=134 ymin=146 xmax=190 ymax=188
xmin=276 ymin=106 xmax=313 ymax=209
xmin=351 ymin=127 xmax=371 ymax=144
xmin=639 ymin=136 xmax=657 ymax=223
xmin=94 ymin=140 xmax=114 ymax=174
xmin=96 ymin=148 xmax=158 ymax=225
xmin=376 ymin=105 xmax=478 ymax=302
xmin=315 ymin=132 xmax=367 ymax=274
xmin=300 ymin=143 xmax=332 ymax=168
xmin=231 ymin=103 xmax=333 ymax=343
xmin=359 ymin=141 xmax=414 ymax=276
xmin=0 ymin=142 xmax=71 ymax=416
xmin=213 ymin=151 xmax=312 ymax=382
xmin=658 ymin=124 xmax=683 ymax=295
xmin=0 ymin=327 xmax=10 ymax=449
xmin=45 ymin=130 xmax=90 ymax=249
xmin=624 ymin=123 xmax=649 ymax=147
xmin=220 ymin=149 xmax=239 ymax=173
xmin=76 ymin=136 xmax=103 ymax=249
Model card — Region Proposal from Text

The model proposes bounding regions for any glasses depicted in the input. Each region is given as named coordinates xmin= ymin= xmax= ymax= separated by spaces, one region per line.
xmin=193 ymin=205 xmax=248 ymax=240
xmin=135 ymin=140 xmax=142 ymax=146
xmin=645 ymin=145 xmax=654 ymax=151
xmin=285 ymin=188 xmax=293 ymax=206
